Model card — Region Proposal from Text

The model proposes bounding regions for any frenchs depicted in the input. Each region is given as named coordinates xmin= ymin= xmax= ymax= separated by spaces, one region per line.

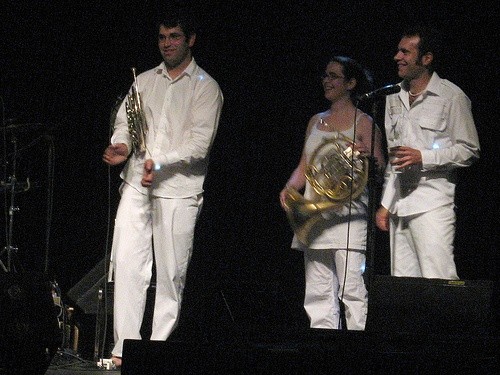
xmin=284 ymin=116 xmax=371 ymax=248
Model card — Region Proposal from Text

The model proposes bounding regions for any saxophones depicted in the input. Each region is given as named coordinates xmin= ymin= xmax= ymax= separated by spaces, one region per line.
xmin=124 ymin=66 xmax=149 ymax=155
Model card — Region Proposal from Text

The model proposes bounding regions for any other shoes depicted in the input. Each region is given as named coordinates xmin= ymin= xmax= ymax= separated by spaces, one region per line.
xmin=109 ymin=355 xmax=123 ymax=368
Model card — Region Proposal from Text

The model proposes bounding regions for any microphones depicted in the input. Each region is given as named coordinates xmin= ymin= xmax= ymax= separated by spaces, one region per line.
xmin=359 ymin=84 xmax=401 ymax=101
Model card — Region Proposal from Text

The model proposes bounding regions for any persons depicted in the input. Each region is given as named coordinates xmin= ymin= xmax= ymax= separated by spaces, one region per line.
xmin=375 ymin=25 xmax=480 ymax=281
xmin=280 ymin=56 xmax=385 ymax=331
xmin=97 ymin=9 xmax=223 ymax=370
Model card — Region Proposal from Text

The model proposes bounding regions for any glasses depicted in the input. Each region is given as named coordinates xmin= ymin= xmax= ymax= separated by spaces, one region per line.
xmin=320 ymin=73 xmax=347 ymax=81
xmin=156 ymin=31 xmax=192 ymax=43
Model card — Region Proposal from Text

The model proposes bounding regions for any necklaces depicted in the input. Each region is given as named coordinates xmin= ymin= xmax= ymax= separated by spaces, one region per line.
xmin=409 ymin=91 xmax=424 ymax=97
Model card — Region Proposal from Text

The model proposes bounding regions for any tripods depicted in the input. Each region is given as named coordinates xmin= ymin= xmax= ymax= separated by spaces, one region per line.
xmin=0 ymin=127 xmax=27 ymax=277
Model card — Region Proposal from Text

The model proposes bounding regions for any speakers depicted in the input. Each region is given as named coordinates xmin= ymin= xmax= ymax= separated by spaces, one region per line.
xmin=66 ymin=252 xmax=500 ymax=375
xmin=0 ymin=271 xmax=64 ymax=375
